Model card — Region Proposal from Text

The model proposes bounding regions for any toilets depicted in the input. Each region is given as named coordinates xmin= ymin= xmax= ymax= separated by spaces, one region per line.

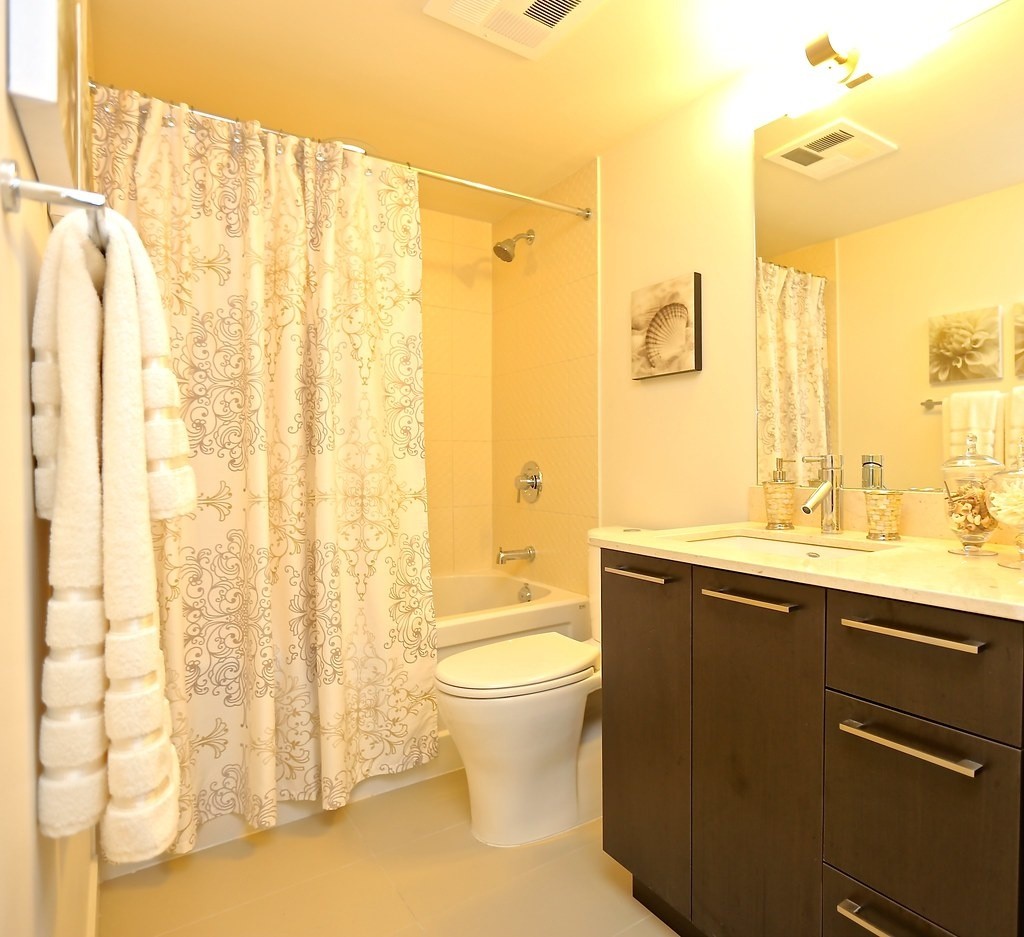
xmin=429 ymin=524 xmax=652 ymax=850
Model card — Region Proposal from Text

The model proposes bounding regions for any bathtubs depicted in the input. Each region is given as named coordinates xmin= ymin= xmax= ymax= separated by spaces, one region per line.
xmin=430 ymin=570 xmax=590 ymax=665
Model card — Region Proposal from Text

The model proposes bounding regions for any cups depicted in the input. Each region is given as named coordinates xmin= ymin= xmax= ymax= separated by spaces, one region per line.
xmin=942 ymin=433 xmax=1007 ymax=557
xmin=984 ymin=436 xmax=1024 ymax=569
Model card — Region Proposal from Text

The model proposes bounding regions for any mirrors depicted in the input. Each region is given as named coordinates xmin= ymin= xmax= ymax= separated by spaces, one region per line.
xmin=754 ymin=0 xmax=1024 ymax=492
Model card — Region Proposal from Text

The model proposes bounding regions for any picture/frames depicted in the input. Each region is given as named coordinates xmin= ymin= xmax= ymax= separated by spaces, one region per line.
xmin=929 ymin=305 xmax=1002 ymax=384
xmin=1013 ymin=301 xmax=1024 ymax=378
xmin=630 ymin=271 xmax=702 ymax=380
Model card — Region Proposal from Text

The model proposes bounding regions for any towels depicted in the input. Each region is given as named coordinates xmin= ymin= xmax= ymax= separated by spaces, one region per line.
xmin=23 ymin=202 xmax=205 ymax=870
xmin=939 ymin=384 xmax=1024 ymax=472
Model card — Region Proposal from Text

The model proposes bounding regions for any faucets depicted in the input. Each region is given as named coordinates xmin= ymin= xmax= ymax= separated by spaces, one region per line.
xmin=797 ymin=453 xmax=845 ymax=534
xmin=861 ymin=453 xmax=944 ymax=493
xmin=495 ymin=546 xmax=535 ymax=565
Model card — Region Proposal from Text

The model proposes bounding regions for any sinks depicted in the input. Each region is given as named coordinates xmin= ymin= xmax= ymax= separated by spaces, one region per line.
xmin=674 ymin=528 xmax=902 ymax=562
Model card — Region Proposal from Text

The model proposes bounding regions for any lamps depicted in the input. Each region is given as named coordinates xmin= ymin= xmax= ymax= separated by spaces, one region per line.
xmin=804 ymin=30 xmax=873 ymax=87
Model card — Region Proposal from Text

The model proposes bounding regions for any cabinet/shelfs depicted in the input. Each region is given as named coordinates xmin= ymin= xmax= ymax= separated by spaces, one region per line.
xmin=824 ymin=586 xmax=1024 ymax=937
xmin=600 ymin=548 xmax=825 ymax=937
